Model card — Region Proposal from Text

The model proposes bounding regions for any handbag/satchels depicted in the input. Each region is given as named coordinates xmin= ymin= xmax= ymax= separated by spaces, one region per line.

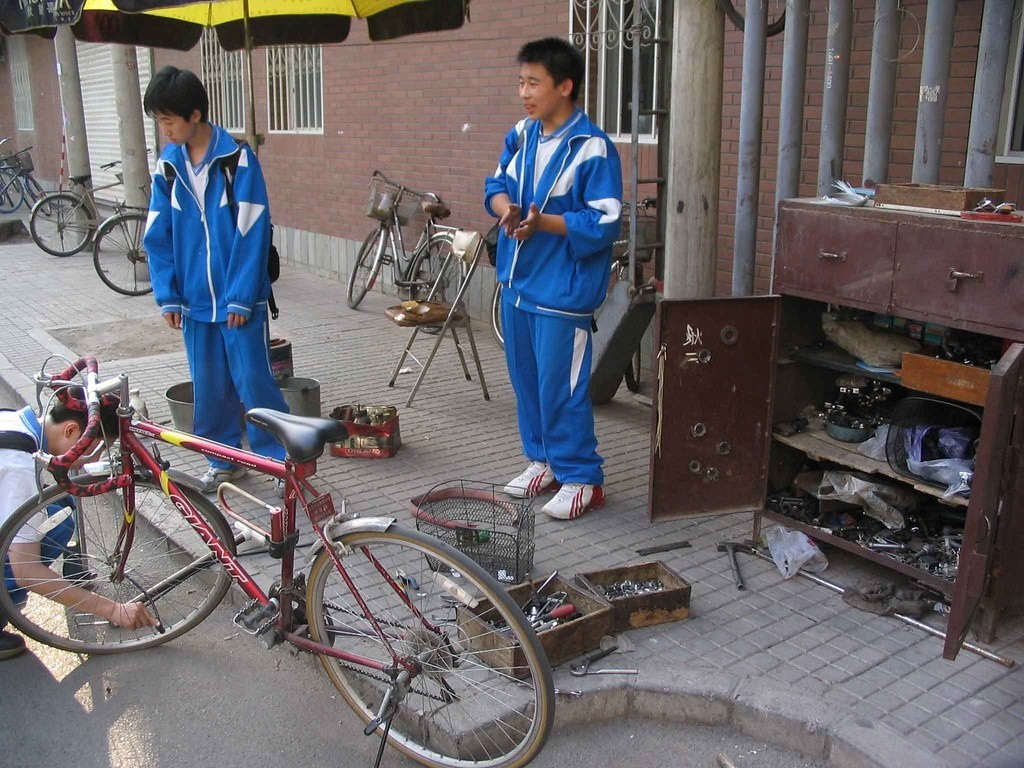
xmin=765 ymin=525 xmax=832 ymax=578
xmin=902 ymin=423 xmax=978 ymax=497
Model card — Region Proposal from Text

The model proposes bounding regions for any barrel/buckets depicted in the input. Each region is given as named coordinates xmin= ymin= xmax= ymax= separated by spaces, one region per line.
xmin=275 ymin=377 xmax=321 ymax=418
xmin=165 ymin=381 xmax=196 ymax=433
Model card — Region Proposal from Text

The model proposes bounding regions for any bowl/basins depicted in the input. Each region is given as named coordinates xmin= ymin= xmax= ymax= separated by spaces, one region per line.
xmin=826 ymin=415 xmax=870 ymax=443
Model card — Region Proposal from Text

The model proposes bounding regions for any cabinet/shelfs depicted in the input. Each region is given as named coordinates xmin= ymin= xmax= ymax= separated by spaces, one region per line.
xmin=650 ymin=189 xmax=1024 ymax=661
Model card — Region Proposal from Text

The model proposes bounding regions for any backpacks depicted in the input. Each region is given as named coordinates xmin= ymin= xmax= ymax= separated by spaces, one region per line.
xmin=165 ymin=137 xmax=279 ymax=287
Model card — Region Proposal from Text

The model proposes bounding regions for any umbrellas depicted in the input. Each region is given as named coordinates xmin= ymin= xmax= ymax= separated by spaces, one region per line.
xmin=0 ymin=0 xmax=475 ymax=159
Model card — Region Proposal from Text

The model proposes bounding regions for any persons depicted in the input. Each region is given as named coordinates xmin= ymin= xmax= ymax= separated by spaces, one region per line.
xmin=143 ymin=67 xmax=304 ymax=500
xmin=484 ymin=36 xmax=624 ymax=522
xmin=0 ymin=389 xmax=160 ymax=664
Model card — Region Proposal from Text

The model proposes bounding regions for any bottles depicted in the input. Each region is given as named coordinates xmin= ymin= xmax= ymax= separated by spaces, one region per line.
xmin=128 ymin=389 xmax=149 ymax=438
xmin=378 ymin=191 xmax=396 ymax=212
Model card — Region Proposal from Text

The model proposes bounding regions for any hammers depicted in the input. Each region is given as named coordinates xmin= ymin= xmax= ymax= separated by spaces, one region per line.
xmin=717 ymin=542 xmax=752 ymax=589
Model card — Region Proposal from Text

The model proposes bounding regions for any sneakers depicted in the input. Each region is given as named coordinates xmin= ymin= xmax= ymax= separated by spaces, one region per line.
xmin=541 ymin=482 xmax=606 ymax=520
xmin=503 ymin=460 xmax=562 ymax=498
xmin=278 ymin=476 xmax=302 ymax=498
xmin=201 ymin=466 xmax=246 ymax=492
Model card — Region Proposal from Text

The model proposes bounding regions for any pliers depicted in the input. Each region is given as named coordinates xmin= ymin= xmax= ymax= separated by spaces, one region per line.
xmin=395 ymin=568 xmax=419 ymax=597
xmin=569 ymin=645 xmax=637 ymax=676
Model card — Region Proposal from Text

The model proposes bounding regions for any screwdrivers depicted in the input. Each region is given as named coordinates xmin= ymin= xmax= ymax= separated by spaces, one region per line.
xmin=529 ymin=604 xmax=574 ymax=623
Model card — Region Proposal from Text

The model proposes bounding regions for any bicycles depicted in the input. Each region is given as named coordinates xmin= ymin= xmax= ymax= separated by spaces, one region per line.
xmin=0 ymin=137 xmax=52 ymax=219
xmin=346 ymin=170 xmax=470 ymax=335
xmin=490 ymin=196 xmax=659 ymax=393
xmin=28 ymin=147 xmax=153 ymax=258
xmin=90 ymin=184 xmax=155 ymax=296
xmin=0 ymin=358 xmax=556 ymax=767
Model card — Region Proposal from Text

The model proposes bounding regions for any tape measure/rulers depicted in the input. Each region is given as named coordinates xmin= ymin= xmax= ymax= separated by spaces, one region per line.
xmin=874 ymin=203 xmax=1023 ymax=223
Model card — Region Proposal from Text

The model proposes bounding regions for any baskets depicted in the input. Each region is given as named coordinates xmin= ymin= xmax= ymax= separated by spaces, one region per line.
xmin=885 ymin=395 xmax=981 ymax=494
xmin=5 ymin=152 xmax=34 ymax=176
xmin=366 ymin=180 xmax=420 ymax=225
xmin=484 ymin=219 xmax=501 ymax=266
xmin=115 ymin=171 xmax=152 ymax=186
xmin=612 ymin=214 xmax=659 ymax=262
xmin=414 ymin=479 xmax=537 ymax=585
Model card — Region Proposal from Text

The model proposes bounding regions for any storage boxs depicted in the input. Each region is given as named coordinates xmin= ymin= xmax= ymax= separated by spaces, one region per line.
xmin=901 ymin=344 xmax=991 ymax=406
xmin=457 ymin=574 xmax=616 ymax=682
xmin=874 ymin=183 xmax=1006 ymax=209
xmin=328 ymin=406 xmax=401 ymax=458
xmin=575 ymin=558 xmax=692 ymax=632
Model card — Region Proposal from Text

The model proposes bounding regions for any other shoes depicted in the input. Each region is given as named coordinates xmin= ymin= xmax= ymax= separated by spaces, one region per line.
xmin=0 ymin=630 xmax=25 ymax=660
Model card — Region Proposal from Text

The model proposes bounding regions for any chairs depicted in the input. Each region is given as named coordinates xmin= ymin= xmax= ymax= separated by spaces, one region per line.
xmin=385 ymin=227 xmax=490 ymax=407
